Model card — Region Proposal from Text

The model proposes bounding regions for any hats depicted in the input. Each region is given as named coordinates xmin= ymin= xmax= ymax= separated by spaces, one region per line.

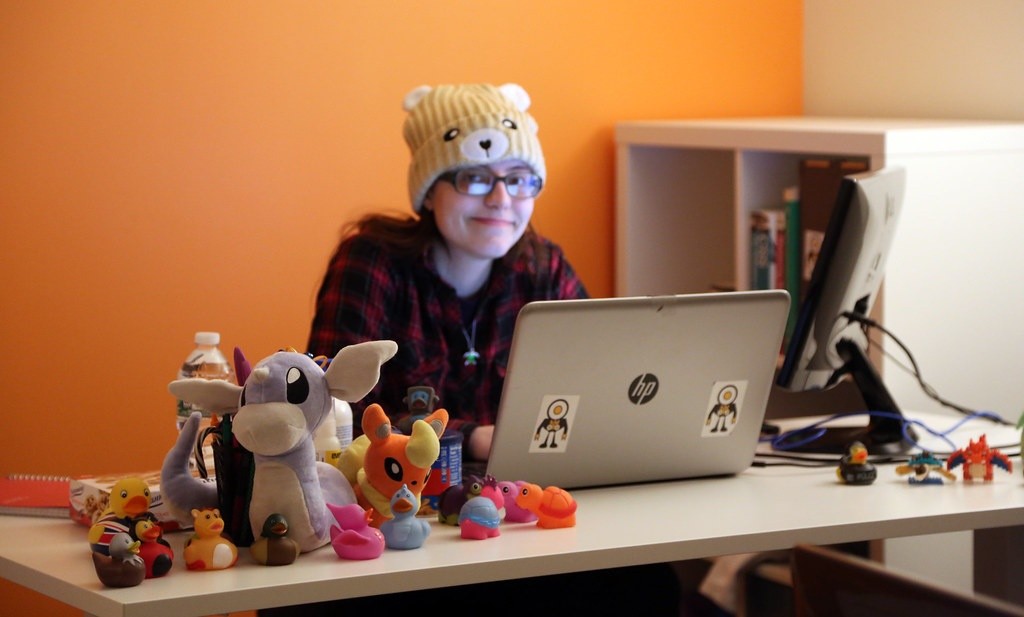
xmin=402 ymin=83 xmax=547 ymax=215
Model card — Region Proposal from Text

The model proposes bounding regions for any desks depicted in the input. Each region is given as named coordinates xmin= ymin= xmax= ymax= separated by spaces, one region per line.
xmin=2 ymin=413 xmax=1024 ymax=617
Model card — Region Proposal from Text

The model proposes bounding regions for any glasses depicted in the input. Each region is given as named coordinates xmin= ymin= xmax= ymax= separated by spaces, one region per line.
xmin=441 ymin=165 xmax=542 ymax=200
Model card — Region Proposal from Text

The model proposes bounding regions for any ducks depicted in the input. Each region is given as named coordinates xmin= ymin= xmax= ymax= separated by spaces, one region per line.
xmin=326 ymin=483 xmax=432 ymax=561
xmin=249 ymin=514 xmax=299 ymax=565
xmin=88 ymin=479 xmax=173 ymax=589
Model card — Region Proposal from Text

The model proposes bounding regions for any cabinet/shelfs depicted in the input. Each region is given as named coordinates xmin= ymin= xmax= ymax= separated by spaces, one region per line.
xmin=612 ymin=115 xmax=1024 ymax=602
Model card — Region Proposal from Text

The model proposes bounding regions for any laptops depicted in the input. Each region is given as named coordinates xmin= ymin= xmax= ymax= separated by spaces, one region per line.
xmin=461 ymin=289 xmax=791 ymax=491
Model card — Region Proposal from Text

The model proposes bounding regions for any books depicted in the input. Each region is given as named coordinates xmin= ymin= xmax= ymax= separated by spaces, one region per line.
xmin=748 ymin=186 xmax=826 ymax=368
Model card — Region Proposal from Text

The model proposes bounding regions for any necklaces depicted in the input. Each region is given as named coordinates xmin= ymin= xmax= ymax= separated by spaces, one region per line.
xmin=462 ymin=321 xmax=481 ymax=368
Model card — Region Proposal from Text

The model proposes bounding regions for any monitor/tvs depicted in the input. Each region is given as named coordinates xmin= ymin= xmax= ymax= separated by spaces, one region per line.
xmin=776 ymin=164 xmax=907 ymax=395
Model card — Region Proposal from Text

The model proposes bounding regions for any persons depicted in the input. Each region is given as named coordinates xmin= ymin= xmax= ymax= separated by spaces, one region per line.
xmin=301 ymin=82 xmax=595 ymax=486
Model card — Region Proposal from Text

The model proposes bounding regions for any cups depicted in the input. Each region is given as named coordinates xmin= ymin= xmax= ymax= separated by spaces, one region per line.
xmin=430 ymin=430 xmax=465 ymax=509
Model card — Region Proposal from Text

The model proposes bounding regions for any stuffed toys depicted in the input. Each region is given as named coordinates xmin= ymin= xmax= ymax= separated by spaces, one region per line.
xmin=159 ymin=340 xmax=398 ymax=560
